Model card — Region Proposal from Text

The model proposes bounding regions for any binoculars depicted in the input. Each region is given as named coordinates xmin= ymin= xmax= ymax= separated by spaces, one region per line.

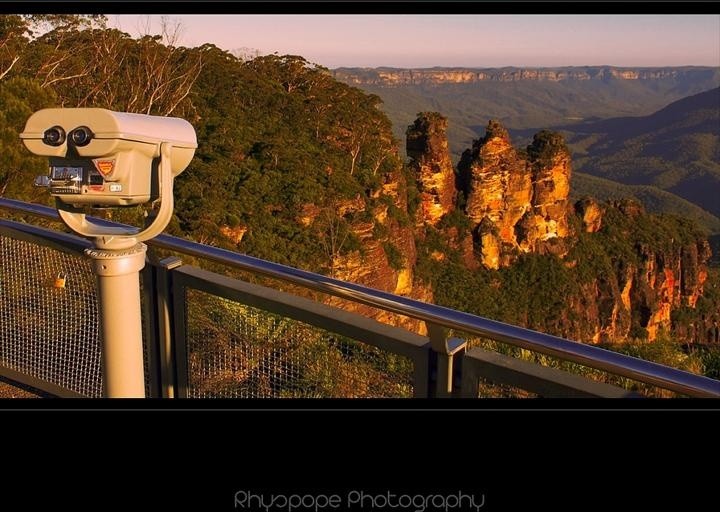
xmin=20 ymin=107 xmax=197 ymax=399
